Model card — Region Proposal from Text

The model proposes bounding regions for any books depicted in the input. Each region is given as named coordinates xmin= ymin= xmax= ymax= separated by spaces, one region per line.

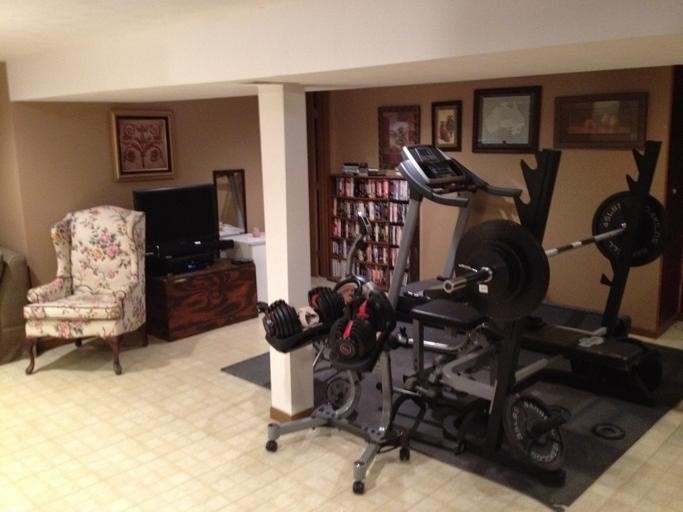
xmin=329 ymin=177 xmax=410 ymax=291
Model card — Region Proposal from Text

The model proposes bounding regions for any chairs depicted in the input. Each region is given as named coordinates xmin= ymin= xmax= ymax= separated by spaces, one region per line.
xmin=23 ymin=205 xmax=148 ymax=373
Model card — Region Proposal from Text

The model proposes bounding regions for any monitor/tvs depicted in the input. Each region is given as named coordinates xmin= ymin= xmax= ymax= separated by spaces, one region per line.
xmin=132 ymin=183 xmax=219 ymax=275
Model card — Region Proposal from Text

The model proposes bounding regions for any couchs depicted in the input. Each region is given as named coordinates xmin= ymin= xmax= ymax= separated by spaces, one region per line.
xmin=0 ymin=245 xmax=37 ymax=366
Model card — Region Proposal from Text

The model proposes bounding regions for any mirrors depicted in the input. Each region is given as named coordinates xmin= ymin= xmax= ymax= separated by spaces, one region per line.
xmin=213 ymin=168 xmax=247 ymax=238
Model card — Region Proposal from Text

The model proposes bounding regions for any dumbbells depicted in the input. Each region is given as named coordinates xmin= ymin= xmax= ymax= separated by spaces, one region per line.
xmin=328 ymin=318 xmax=375 ymax=360
xmin=263 ymin=286 xmax=344 ymax=337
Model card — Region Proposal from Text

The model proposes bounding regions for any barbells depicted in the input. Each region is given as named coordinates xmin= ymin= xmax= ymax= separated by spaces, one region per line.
xmin=444 ymin=191 xmax=668 ymax=322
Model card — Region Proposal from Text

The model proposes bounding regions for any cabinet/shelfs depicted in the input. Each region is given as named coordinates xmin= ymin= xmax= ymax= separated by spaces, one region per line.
xmin=221 ymin=232 xmax=269 ymax=304
xmin=328 ymin=173 xmax=420 ymax=291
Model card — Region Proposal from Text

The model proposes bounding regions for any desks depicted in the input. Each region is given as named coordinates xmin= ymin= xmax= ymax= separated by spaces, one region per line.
xmin=140 ymin=258 xmax=259 ymax=342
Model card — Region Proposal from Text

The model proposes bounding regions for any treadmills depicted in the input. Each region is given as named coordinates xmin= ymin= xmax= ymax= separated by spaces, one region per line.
xmin=386 ymin=145 xmax=640 ymax=372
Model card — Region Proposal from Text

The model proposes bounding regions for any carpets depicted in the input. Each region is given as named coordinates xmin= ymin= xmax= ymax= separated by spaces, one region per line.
xmin=221 ymin=321 xmax=683 ymax=512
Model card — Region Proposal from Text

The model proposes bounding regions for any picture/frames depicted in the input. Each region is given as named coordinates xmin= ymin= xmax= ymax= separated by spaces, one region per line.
xmin=472 ymin=85 xmax=542 ymax=155
xmin=377 ymin=105 xmax=421 ymax=169
xmin=553 ymin=91 xmax=648 ymax=151
xmin=109 ymin=108 xmax=176 ymax=183
xmin=431 ymin=100 xmax=462 ymax=152
